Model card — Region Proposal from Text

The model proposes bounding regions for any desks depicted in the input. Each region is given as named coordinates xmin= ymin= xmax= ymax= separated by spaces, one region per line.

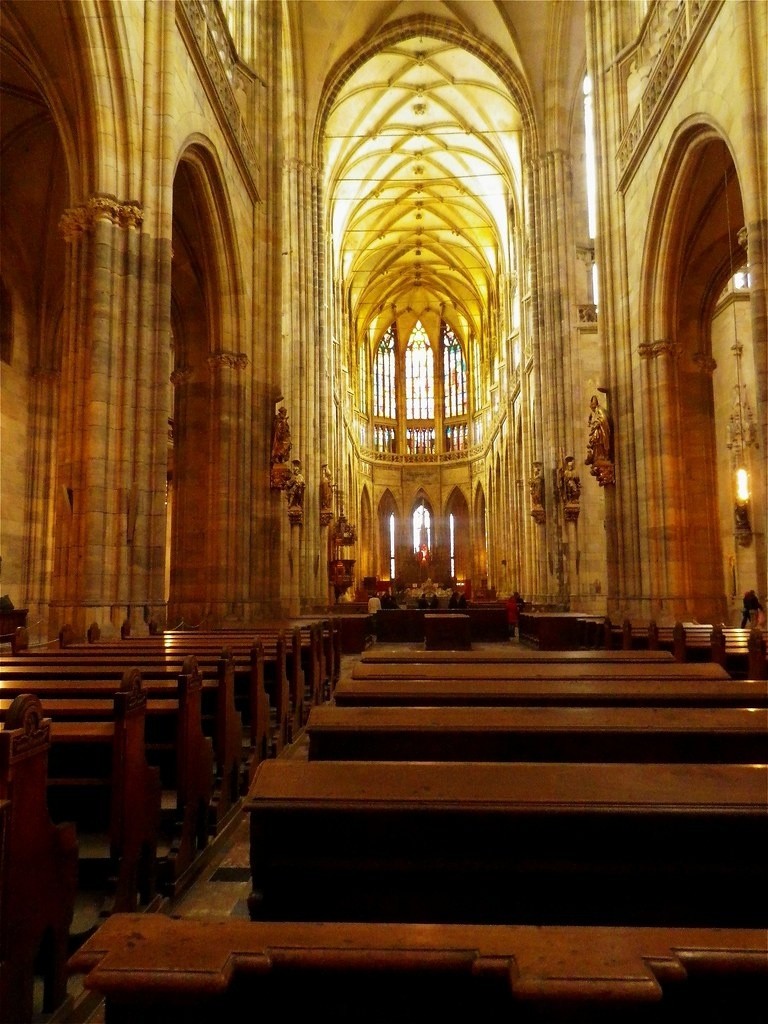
xmin=377 ymin=609 xmax=510 ymax=644
xmin=424 ymin=614 xmax=471 ymax=650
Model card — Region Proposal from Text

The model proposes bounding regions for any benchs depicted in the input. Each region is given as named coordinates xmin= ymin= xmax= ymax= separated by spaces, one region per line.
xmin=0 ymin=612 xmax=768 ymax=1024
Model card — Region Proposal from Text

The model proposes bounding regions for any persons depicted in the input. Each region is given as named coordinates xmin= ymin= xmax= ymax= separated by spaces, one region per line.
xmin=529 ymin=396 xmax=613 ymax=511
xmin=271 ymin=407 xmax=334 ymax=512
xmin=741 ymin=590 xmax=764 ymax=629
xmin=368 ymin=590 xmax=524 ymax=637
